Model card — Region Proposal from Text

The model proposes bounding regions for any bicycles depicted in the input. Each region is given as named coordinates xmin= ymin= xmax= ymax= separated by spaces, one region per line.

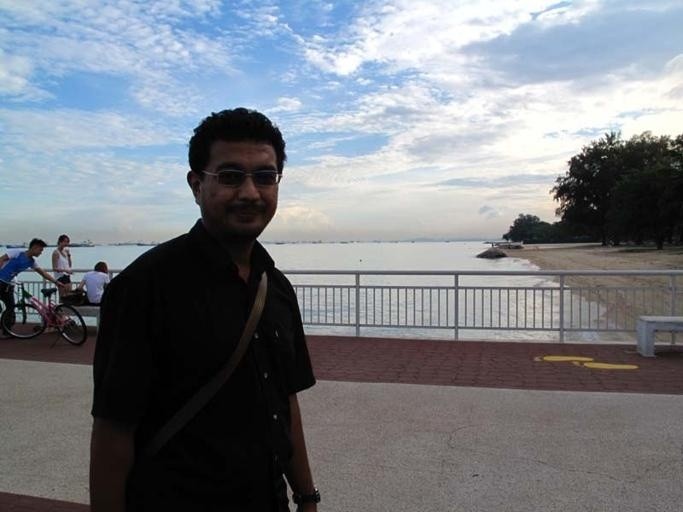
xmin=0 ymin=281 xmax=88 ymax=345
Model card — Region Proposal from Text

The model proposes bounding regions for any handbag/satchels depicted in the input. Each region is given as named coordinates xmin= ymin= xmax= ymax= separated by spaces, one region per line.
xmin=62 ymin=289 xmax=87 ymax=305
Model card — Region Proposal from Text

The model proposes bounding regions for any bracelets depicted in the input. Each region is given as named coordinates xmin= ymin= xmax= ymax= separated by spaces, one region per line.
xmin=292 ymin=486 xmax=320 ymax=505
xmin=55 ymin=281 xmax=58 ymax=283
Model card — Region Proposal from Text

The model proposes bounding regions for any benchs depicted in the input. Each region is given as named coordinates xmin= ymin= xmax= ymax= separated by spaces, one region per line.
xmin=639 ymin=314 xmax=683 ymax=359
xmin=54 ymin=304 xmax=100 ymax=334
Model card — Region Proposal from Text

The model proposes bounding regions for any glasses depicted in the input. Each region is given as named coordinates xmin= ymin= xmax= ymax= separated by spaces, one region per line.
xmin=202 ymin=167 xmax=282 ymax=186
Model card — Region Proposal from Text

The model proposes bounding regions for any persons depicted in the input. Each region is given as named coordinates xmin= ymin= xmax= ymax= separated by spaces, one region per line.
xmin=78 ymin=262 xmax=110 ymax=305
xmin=90 ymin=110 xmax=321 ymax=511
xmin=0 ymin=238 xmax=64 ymax=339
xmin=52 ymin=234 xmax=72 ymax=304
